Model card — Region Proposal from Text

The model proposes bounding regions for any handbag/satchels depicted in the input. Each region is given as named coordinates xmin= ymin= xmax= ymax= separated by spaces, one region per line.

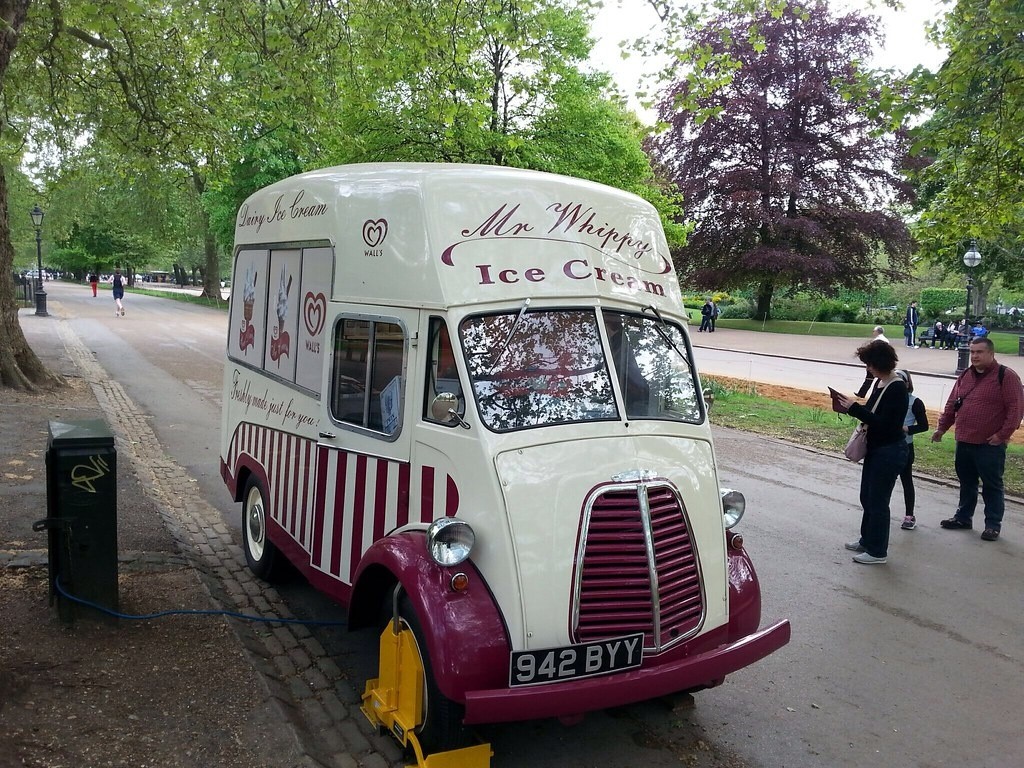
xmin=845 ymin=542 xmax=867 ymax=552
xmin=845 ymin=422 xmax=867 ymax=461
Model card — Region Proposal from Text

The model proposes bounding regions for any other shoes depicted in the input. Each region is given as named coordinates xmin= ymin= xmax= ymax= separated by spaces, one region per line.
xmin=115 ymin=312 xmax=119 ymax=318
xmin=854 ymin=392 xmax=865 ymax=398
xmin=121 ymin=307 xmax=125 ymax=316
xmin=906 ymin=346 xmax=960 ymax=351
xmin=93 ymin=296 xmax=97 ymax=298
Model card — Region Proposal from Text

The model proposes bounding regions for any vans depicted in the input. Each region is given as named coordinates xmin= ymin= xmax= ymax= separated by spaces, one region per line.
xmin=217 ymin=160 xmax=790 ymax=747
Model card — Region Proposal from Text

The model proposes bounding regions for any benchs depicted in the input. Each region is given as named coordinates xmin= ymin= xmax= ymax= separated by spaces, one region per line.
xmin=918 ymin=327 xmax=990 ymax=348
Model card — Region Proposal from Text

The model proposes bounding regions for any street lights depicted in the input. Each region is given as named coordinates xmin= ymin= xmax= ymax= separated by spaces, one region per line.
xmin=957 ymin=238 xmax=981 ymax=376
xmin=29 ymin=203 xmax=49 ymax=316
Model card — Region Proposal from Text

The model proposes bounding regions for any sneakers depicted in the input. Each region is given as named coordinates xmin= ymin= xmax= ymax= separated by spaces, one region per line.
xmin=901 ymin=516 xmax=916 ymax=529
xmin=853 ymin=552 xmax=888 ymax=564
xmin=981 ymin=527 xmax=1000 ymax=540
xmin=941 ymin=518 xmax=972 ymax=529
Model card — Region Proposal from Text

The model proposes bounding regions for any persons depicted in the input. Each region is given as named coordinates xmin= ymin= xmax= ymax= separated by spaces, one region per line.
xmin=697 ymin=299 xmax=717 ymax=333
xmin=894 ymin=369 xmax=929 ymax=530
xmin=904 ymin=301 xmax=920 ymax=348
xmin=107 ymin=268 xmax=125 ymax=318
xmin=931 ymin=337 xmax=1024 ymax=541
xmin=853 ymin=326 xmax=890 ymax=399
xmin=90 ymin=272 xmax=99 ymax=297
xmin=837 ymin=340 xmax=909 ymax=563
xmin=929 ymin=319 xmax=986 ymax=351
